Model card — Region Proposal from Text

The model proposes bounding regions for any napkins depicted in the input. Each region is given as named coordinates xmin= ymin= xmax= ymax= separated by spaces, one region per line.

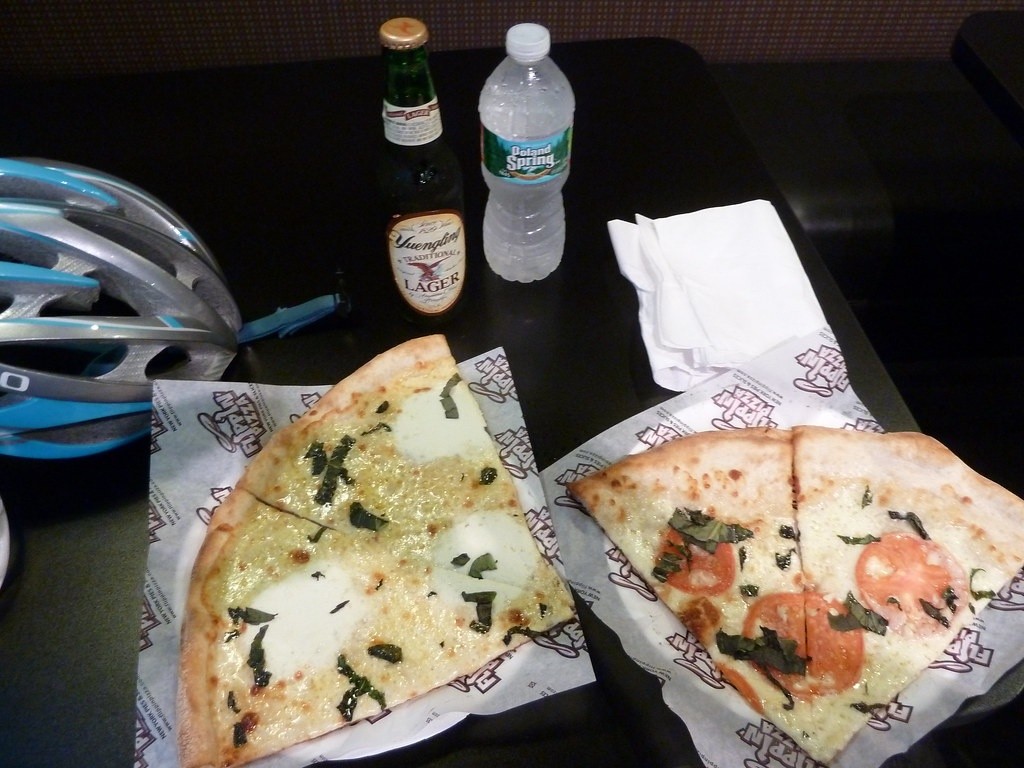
xmin=607 ymin=199 xmax=831 ymax=392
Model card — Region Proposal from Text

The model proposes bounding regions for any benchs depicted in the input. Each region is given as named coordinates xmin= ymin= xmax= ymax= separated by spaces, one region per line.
xmin=0 ymin=1 xmax=1024 ymax=234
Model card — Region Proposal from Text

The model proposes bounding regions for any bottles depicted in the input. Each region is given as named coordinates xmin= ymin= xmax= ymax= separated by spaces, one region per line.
xmin=477 ymin=23 xmax=576 ymax=284
xmin=374 ymin=18 xmax=472 ymax=326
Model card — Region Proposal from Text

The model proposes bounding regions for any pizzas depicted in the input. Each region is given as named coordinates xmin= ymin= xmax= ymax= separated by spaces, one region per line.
xmin=178 ymin=333 xmax=575 ymax=768
xmin=567 ymin=428 xmax=1024 ymax=765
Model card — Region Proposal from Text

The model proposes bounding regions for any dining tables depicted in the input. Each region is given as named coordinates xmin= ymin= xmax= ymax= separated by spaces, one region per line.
xmin=0 ymin=36 xmax=937 ymax=768
